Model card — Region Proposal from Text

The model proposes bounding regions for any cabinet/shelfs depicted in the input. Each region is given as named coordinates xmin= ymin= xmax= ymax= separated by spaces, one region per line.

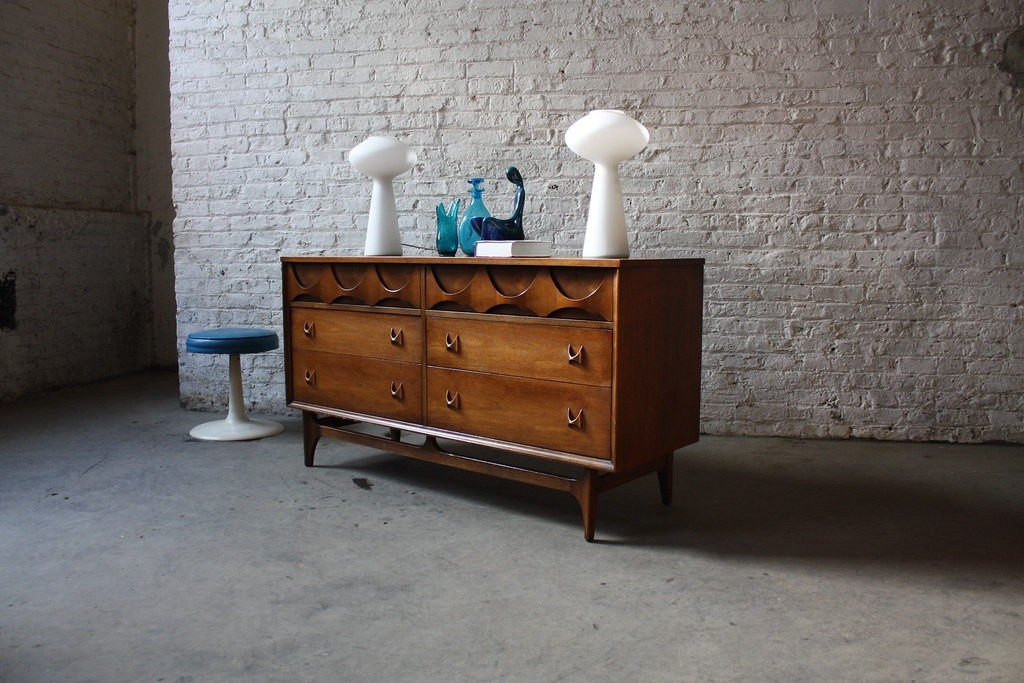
xmin=280 ymin=254 xmax=704 ymax=542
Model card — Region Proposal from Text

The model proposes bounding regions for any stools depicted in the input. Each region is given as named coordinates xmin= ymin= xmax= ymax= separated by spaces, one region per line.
xmin=186 ymin=328 xmax=285 ymax=441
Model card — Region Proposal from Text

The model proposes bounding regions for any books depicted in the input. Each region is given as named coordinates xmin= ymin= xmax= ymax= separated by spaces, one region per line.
xmin=473 ymin=240 xmax=552 ymax=258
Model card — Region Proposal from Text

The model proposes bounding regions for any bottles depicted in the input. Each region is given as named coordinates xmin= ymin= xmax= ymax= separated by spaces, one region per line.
xmin=458 ymin=175 xmax=493 ymax=256
